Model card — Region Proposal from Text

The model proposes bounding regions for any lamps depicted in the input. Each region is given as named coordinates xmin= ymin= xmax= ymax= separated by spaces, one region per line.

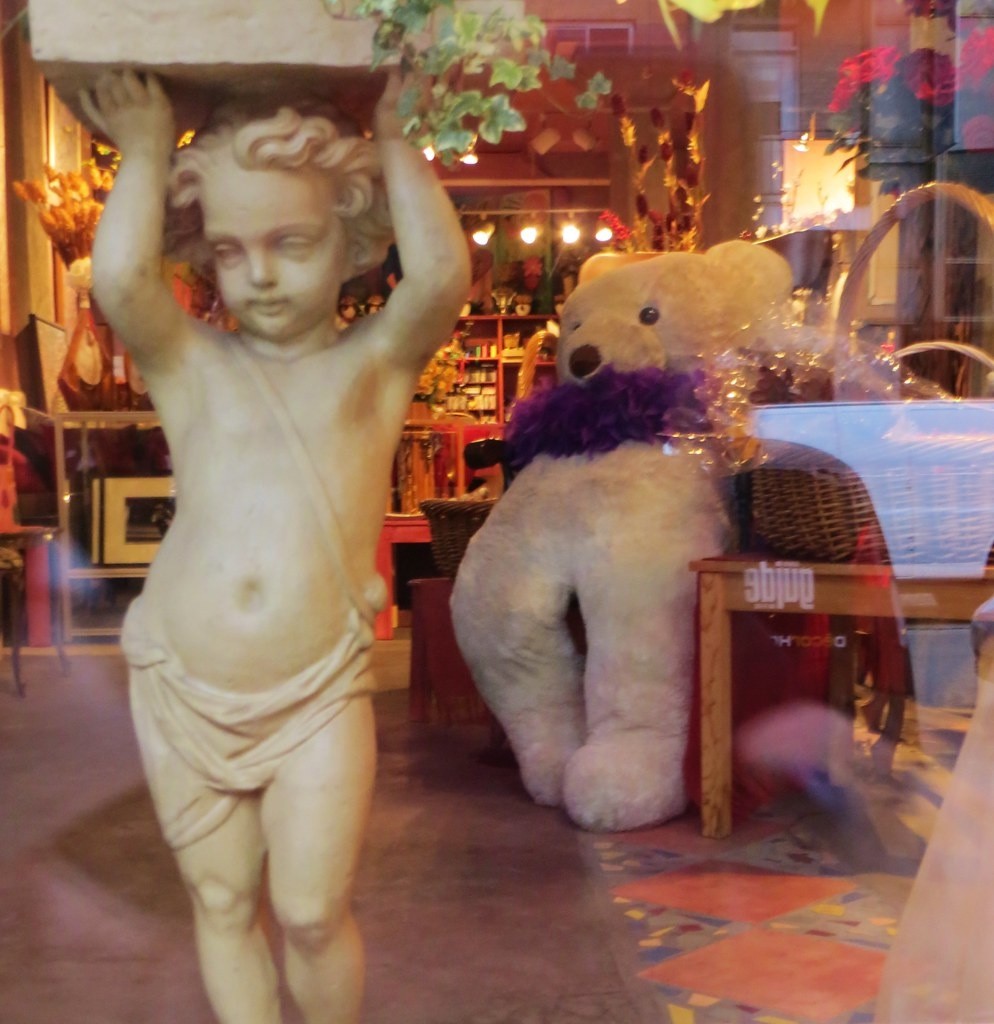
xmin=562 ymin=221 xmax=581 ymax=243
xmin=595 ymin=218 xmax=612 ymax=242
xmin=472 ymin=220 xmax=496 ymax=246
xmin=519 ymin=222 xmax=545 ymax=245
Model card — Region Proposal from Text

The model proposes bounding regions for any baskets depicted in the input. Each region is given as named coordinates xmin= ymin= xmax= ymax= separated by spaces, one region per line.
xmin=751 ymin=179 xmax=993 ymax=564
xmin=415 ymin=330 xmax=559 ymax=577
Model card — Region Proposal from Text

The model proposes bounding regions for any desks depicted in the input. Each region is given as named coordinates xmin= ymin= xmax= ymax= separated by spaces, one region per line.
xmin=684 ymin=558 xmax=994 ymax=839
xmin=373 ymin=518 xmax=431 ymax=642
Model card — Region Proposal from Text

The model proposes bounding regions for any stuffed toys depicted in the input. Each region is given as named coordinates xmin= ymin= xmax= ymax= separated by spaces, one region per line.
xmin=450 ymin=240 xmax=797 ymax=834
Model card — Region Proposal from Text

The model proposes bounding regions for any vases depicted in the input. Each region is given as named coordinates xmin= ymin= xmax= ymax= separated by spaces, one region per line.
xmin=59 ymin=290 xmax=118 ymax=408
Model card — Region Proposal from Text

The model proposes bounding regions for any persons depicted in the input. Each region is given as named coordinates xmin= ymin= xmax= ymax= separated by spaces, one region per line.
xmin=78 ymin=55 xmax=473 ymax=1023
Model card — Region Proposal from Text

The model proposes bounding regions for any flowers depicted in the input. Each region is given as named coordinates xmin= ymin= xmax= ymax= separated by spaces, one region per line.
xmin=10 ymin=163 xmax=105 ymax=287
xmin=823 ymin=0 xmax=994 ymax=156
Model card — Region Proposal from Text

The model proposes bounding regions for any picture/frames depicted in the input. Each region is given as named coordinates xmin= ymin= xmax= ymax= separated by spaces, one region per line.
xmin=29 ymin=314 xmax=68 ymax=416
xmin=103 ymin=477 xmax=175 ymax=563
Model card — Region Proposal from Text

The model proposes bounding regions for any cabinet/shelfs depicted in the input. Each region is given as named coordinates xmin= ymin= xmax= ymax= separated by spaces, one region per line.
xmin=451 ymin=314 xmax=561 ymax=424
